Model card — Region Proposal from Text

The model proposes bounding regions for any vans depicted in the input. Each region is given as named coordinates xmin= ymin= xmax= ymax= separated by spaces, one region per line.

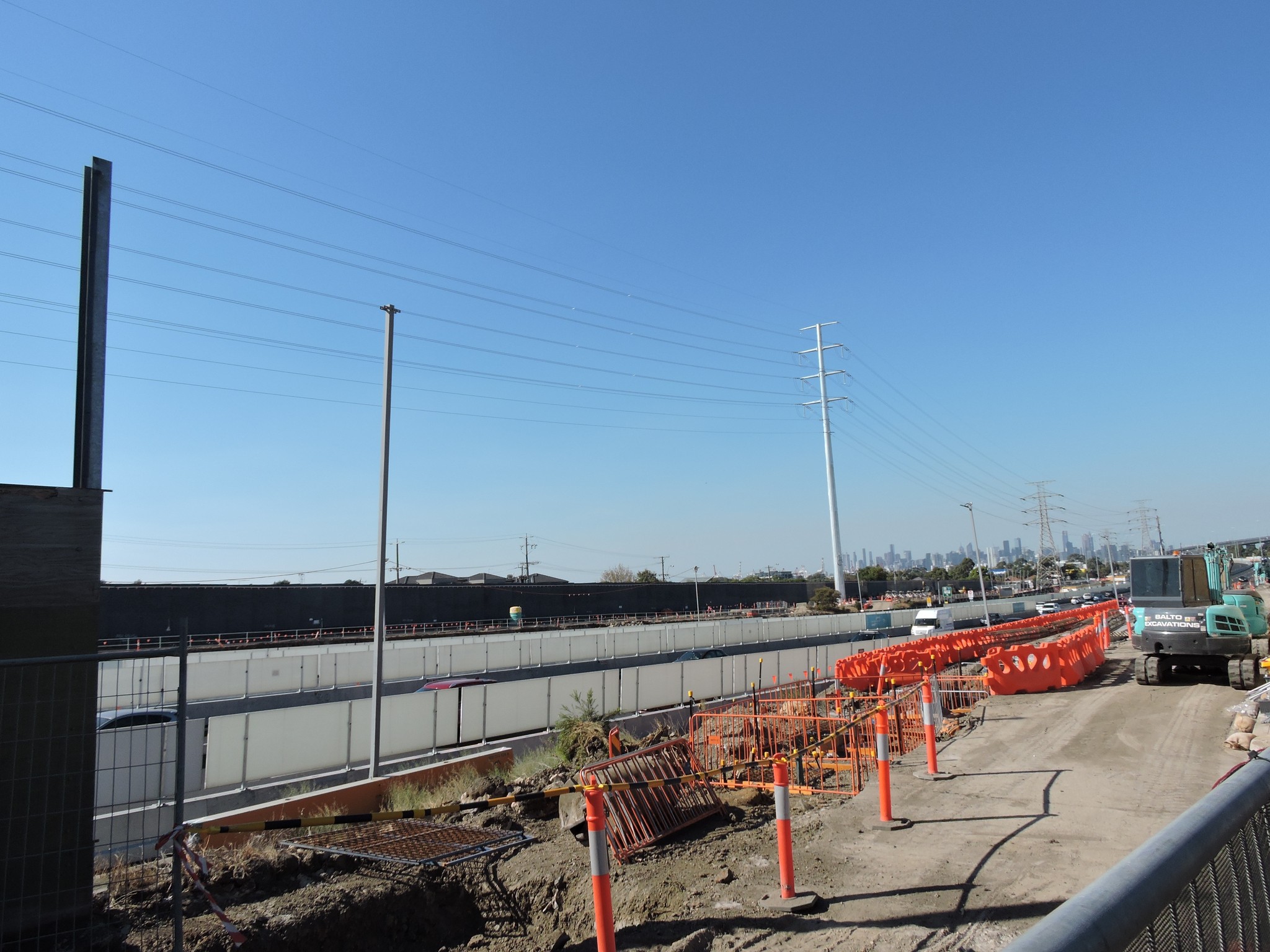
xmin=910 ymin=608 xmax=955 ymax=636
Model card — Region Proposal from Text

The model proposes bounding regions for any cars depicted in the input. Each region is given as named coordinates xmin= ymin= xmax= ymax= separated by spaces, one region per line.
xmin=1042 ymin=602 xmax=1062 ymax=614
xmin=981 ymin=613 xmax=1004 ymax=627
xmin=1006 ymin=618 xmax=1024 ymax=623
xmin=1036 ymin=602 xmax=1046 ymax=610
xmin=1081 ymin=590 xmax=1129 ymax=608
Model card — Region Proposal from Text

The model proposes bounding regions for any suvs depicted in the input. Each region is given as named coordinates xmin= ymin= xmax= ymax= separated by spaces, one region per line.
xmin=1071 ymin=596 xmax=1086 ymax=604
xmin=847 ymin=630 xmax=889 ymax=642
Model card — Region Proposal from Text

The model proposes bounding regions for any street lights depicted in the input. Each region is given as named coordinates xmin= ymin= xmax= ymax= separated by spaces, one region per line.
xmin=961 ymin=502 xmax=989 ymax=626
xmin=1101 ymin=536 xmax=1119 ymax=604
xmin=694 ymin=566 xmax=700 ymax=621
xmin=856 ymin=571 xmax=864 ymax=610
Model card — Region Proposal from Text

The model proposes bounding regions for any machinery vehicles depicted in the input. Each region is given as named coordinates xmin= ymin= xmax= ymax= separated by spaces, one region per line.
xmin=1128 ymin=543 xmax=1267 ymax=691
